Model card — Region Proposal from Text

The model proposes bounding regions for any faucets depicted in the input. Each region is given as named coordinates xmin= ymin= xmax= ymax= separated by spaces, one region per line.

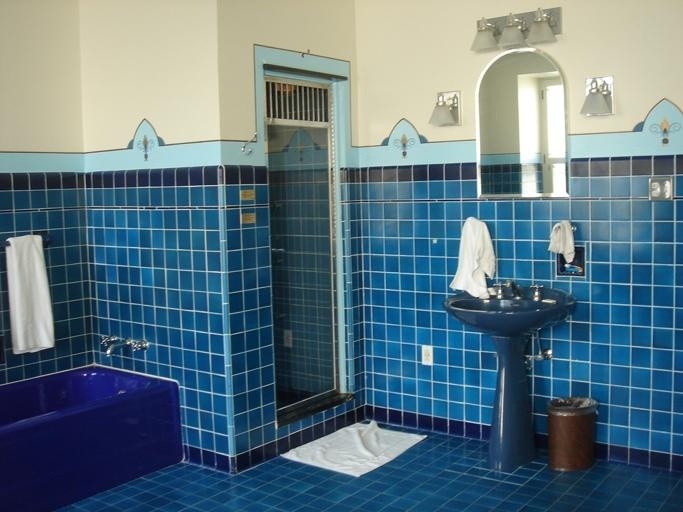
xmin=504 ymin=278 xmax=521 ymax=298
xmin=101 ymin=336 xmax=131 ymax=357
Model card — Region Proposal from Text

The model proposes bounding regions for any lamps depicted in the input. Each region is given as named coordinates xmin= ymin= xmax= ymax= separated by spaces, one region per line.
xmin=579 ymin=76 xmax=614 ymax=118
xmin=428 ymin=91 xmax=462 ymax=128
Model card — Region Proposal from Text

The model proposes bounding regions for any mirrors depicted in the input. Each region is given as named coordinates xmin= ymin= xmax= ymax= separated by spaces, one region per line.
xmin=473 ymin=48 xmax=569 ymax=198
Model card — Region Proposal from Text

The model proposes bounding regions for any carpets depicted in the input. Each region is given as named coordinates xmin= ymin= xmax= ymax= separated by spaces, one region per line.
xmin=280 ymin=419 xmax=428 ymax=479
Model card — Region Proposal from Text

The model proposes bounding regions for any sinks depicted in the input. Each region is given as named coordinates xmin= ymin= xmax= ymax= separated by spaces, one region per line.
xmin=442 ymin=289 xmax=577 ymax=333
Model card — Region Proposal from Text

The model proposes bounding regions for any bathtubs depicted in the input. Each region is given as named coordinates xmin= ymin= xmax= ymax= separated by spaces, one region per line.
xmin=0 ymin=365 xmax=183 ymax=511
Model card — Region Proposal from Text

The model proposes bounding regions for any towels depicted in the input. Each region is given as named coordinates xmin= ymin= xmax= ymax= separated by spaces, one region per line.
xmin=448 ymin=215 xmax=497 ymax=300
xmin=4 ymin=234 xmax=57 ymax=356
xmin=548 ymin=220 xmax=576 ymax=263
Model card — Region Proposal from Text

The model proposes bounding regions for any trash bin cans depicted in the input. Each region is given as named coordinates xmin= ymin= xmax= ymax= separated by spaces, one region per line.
xmin=547 ymin=398 xmax=599 ymax=472
